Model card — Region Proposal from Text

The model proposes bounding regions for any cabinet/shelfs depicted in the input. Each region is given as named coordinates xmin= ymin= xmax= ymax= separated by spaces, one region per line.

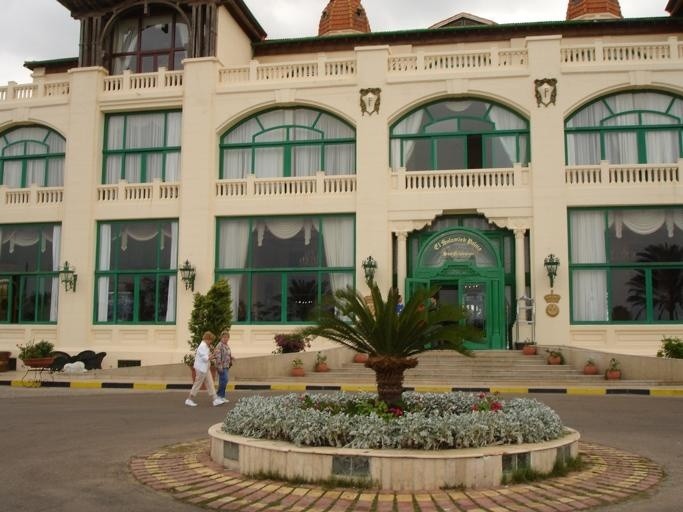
xmin=22 ymin=366 xmax=56 ymax=389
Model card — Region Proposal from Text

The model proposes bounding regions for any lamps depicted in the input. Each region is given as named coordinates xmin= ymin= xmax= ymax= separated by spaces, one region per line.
xmin=544 ymin=253 xmax=560 ymax=293
xmin=176 ymin=259 xmax=197 ymax=292
xmin=362 ymin=256 xmax=377 ymax=292
xmin=57 ymin=260 xmax=78 ymax=294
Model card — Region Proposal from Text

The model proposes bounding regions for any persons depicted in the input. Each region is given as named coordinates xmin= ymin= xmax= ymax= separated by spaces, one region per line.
xmin=212 ymin=329 xmax=236 ymax=404
xmin=395 ymin=293 xmax=403 ymax=315
xmin=184 ymin=330 xmax=225 ymax=406
xmin=428 ymin=295 xmax=444 ymax=350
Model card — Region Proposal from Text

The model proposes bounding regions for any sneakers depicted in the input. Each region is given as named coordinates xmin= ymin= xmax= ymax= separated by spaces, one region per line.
xmin=212 ymin=397 xmax=229 ymax=407
xmin=185 ymin=398 xmax=198 ymax=406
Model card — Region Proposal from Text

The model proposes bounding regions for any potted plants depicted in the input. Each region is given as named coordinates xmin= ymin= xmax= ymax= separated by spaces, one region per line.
xmin=16 ymin=340 xmax=54 ymax=367
xmin=290 ymin=351 xmax=369 ymax=377
xmin=182 ymin=277 xmax=234 ymax=391
xmin=521 ymin=338 xmax=620 ymax=381
